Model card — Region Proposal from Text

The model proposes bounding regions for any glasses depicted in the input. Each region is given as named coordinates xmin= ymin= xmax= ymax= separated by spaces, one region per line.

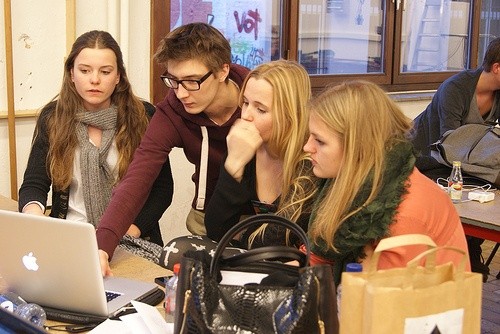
xmin=159 ymin=69 xmax=214 ymax=91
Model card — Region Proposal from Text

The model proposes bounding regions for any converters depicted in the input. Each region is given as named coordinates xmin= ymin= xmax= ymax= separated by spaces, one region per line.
xmin=468 ymin=191 xmax=495 ymax=203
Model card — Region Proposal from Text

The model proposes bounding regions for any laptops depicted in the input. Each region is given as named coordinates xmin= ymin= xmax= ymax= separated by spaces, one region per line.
xmin=0 ymin=210 xmax=158 ymax=318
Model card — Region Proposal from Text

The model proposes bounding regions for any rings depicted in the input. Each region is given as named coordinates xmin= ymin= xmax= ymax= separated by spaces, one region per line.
xmin=241 ymin=124 xmax=250 ymax=130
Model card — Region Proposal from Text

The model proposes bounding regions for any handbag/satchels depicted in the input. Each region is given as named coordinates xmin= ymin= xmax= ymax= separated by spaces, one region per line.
xmin=342 ymin=234 xmax=483 ymax=334
xmin=175 ymin=214 xmax=340 ymax=334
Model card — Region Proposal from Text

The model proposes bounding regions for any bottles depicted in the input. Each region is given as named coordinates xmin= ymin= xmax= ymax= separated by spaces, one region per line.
xmin=448 ymin=160 xmax=463 ymax=204
xmin=165 ymin=264 xmax=181 ymax=323
xmin=336 ymin=262 xmax=363 ymax=321
xmin=0 ymin=286 xmax=47 ymax=328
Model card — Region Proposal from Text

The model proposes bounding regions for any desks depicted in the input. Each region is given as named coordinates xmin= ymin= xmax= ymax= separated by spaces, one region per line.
xmin=452 ymin=189 xmax=500 ymax=243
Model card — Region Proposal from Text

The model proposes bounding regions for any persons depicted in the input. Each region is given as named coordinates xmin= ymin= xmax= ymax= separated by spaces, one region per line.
xmin=411 ymin=38 xmax=500 ymax=283
xmin=248 ymin=80 xmax=471 ymax=294
xmin=204 ymin=59 xmax=327 ymax=250
xmin=18 ymin=30 xmax=174 ymax=247
xmin=95 ymin=22 xmax=252 ymax=278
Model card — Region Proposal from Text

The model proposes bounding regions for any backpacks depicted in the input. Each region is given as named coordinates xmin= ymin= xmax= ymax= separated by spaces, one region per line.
xmin=429 ymin=122 xmax=499 ymax=188
